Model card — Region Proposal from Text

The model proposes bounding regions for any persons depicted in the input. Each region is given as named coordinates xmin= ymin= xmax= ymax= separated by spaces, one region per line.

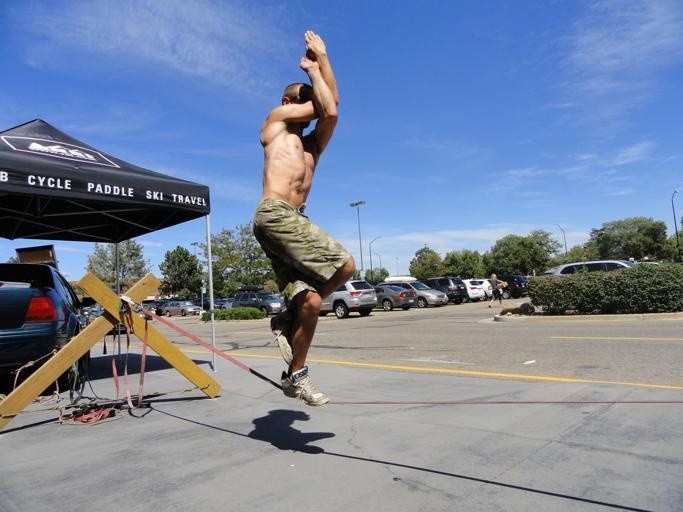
xmin=488 ymin=273 xmax=503 ymax=308
xmin=252 ymin=30 xmax=355 ymax=407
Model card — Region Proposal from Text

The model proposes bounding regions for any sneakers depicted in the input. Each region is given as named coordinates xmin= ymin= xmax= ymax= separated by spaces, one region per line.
xmin=270 ymin=314 xmax=293 ymax=364
xmin=489 ymin=304 xmax=492 ymax=307
xmin=280 ymin=366 xmax=330 ymax=406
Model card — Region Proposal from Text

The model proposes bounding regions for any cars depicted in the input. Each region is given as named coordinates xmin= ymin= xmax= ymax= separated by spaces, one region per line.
xmin=88 ymin=290 xmax=288 ymax=332
xmin=320 ymin=276 xmax=530 ymax=319
xmin=553 ymin=259 xmax=635 ymax=275
xmin=0 ymin=263 xmax=95 ymax=389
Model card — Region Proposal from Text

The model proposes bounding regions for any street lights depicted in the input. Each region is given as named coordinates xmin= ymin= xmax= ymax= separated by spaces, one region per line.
xmin=555 ymin=223 xmax=567 ymax=253
xmin=369 ymin=236 xmax=382 ymax=283
xmin=671 ymin=190 xmax=682 ymax=262
xmin=350 ymin=201 xmax=364 ymax=271
